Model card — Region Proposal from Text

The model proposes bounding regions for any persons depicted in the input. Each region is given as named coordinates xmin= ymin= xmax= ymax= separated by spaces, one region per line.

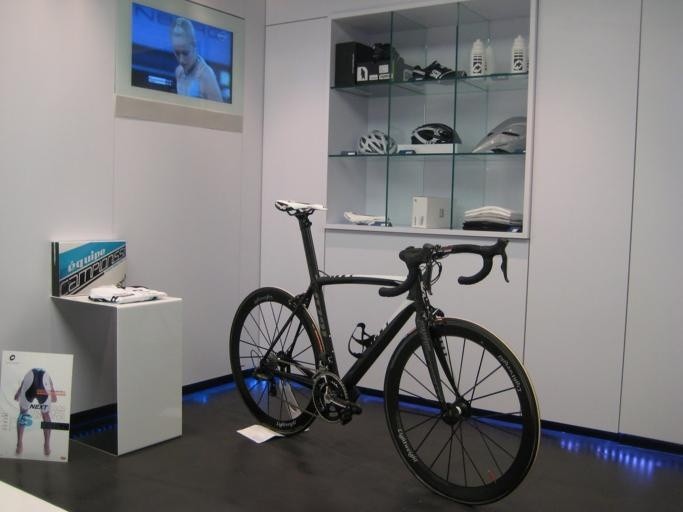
xmin=171 ymin=17 xmax=223 ymax=102
xmin=12 ymin=367 xmax=56 ymax=456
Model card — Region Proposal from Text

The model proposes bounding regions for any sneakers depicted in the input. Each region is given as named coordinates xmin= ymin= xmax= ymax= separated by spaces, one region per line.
xmin=413 ymin=61 xmax=466 ymax=80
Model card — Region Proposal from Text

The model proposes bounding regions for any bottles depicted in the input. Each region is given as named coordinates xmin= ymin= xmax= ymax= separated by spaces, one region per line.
xmin=469 ymin=36 xmax=495 ymax=81
xmin=511 ymin=33 xmax=528 ymax=80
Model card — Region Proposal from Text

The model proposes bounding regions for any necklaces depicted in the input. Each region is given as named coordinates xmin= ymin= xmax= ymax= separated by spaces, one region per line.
xmin=180 ymin=56 xmax=200 ymax=81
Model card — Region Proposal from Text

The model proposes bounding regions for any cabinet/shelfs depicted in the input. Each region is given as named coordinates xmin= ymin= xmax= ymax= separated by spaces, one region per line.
xmin=320 ymin=0 xmax=540 ymax=241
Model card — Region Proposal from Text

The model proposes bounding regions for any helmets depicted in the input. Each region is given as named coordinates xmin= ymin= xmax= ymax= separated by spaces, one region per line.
xmin=358 ymin=130 xmax=397 ymax=154
xmin=411 ymin=124 xmax=461 ymax=144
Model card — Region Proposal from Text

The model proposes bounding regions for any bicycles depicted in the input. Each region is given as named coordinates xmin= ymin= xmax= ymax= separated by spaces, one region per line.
xmin=227 ymin=196 xmax=542 ymax=508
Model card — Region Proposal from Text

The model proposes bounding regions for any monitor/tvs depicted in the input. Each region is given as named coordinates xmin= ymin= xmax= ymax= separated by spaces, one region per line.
xmin=114 ymin=0 xmax=246 ymax=117
